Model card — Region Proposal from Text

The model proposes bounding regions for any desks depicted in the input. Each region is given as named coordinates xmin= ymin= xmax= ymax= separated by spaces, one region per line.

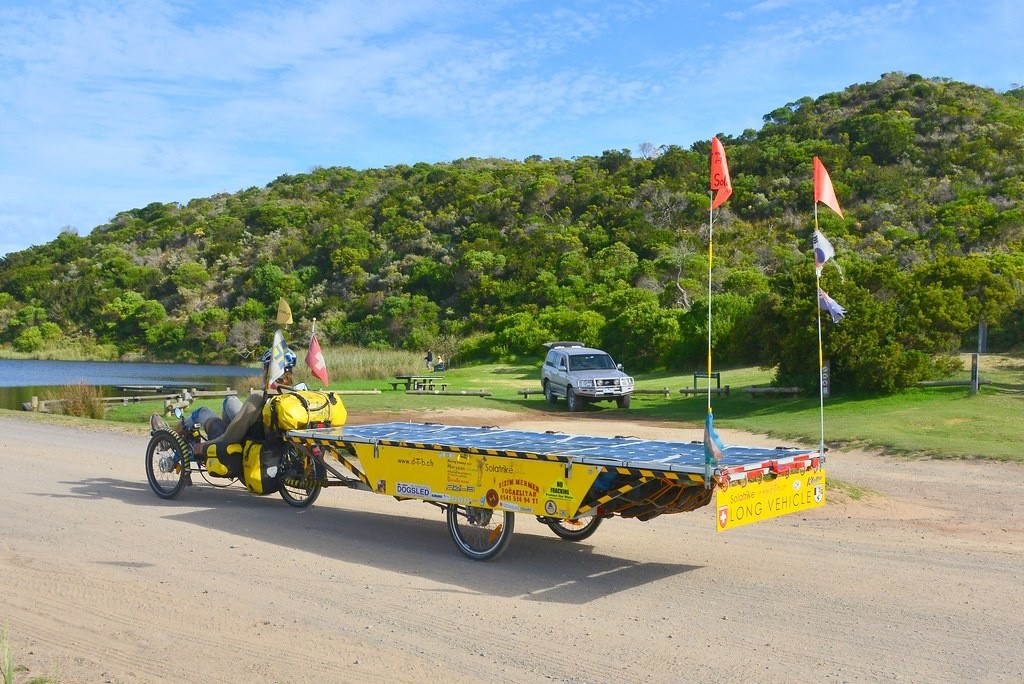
xmin=396 ymin=376 xmax=443 ymax=391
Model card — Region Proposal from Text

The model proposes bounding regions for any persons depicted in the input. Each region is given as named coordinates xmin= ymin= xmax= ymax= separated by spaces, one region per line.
xmin=433 ymin=356 xmax=443 ymax=372
xmin=150 ymin=347 xmax=296 ymax=458
xmin=423 ymin=348 xmax=432 ymax=373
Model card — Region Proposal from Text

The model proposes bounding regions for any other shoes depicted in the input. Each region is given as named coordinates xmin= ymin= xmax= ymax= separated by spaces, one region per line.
xmin=151 ymin=413 xmax=170 ymax=449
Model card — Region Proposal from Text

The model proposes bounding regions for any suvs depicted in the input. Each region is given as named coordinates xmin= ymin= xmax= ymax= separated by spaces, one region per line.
xmin=540 ymin=341 xmax=635 ymax=413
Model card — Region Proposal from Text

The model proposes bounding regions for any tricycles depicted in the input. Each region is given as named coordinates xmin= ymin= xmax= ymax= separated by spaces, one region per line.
xmin=144 ymin=368 xmax=346 ymax=508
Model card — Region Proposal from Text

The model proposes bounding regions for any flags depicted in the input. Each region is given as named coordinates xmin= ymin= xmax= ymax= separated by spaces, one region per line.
xmin=306 ymin=334 xmax=329 ymax=387
xmin=265 ymin=324 xmax=296 ymax=392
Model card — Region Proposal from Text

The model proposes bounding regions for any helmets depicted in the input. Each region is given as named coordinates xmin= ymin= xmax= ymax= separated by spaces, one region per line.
xmin=257 ymin=346 xmax=296 ymax=368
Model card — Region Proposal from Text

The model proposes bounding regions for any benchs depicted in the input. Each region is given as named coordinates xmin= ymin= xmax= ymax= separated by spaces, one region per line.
xmin=388 ymin=381 xmax=423 ymax=391
xmin=418 ymin=383 xmax=451 ymax=391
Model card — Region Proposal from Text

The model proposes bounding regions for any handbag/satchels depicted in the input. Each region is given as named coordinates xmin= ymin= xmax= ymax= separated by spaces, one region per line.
xmin=203 ymin=437 xmax=285 ymax=496
